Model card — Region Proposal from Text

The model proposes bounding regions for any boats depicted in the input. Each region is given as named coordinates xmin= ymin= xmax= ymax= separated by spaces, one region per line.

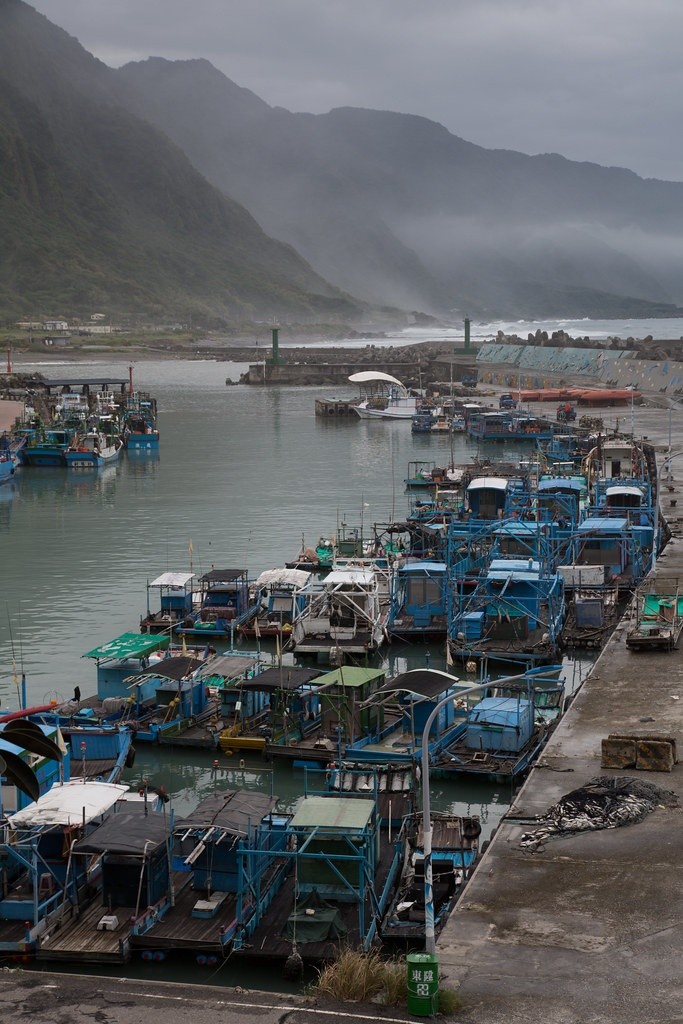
xmin=0 ymin=438 xmax=671 ymax=965
xmin=314 ymin=367 xmax=604 ymax=440
xmin=0 ymin=371 xmax=161 ymax=485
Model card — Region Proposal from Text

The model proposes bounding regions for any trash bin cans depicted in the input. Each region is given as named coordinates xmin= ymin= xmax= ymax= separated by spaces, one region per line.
xmin=406 ymin=954 xmax=439 ymax=1016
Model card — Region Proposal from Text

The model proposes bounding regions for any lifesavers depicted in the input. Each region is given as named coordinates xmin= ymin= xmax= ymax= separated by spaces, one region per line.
xmin=282 ymin=638 xmax=296 ymax=651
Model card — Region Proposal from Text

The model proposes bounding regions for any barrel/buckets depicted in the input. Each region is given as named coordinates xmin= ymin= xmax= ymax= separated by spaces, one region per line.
xmin=406 ymin=952 xmax=439 ymax=1017
